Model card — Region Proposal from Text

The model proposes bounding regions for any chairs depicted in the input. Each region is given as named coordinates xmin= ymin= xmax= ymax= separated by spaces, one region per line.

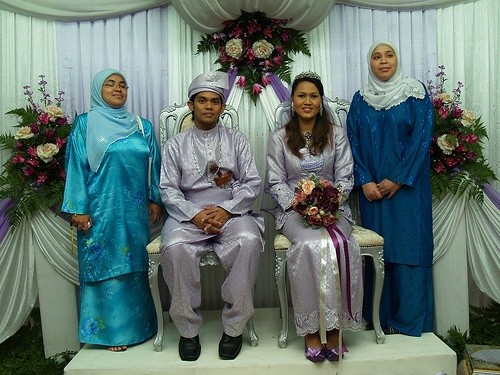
xmin=274 ymin=96 xmax=385 ymax=348
xmin=147 ymin=102 xmax=259 ymax=352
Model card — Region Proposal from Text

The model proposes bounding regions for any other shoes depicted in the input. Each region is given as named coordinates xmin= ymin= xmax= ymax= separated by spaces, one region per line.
xmin=324 ymin=330 xmax=344 ymax=363
xmin=304 ymin=335 xmax=325 ymax=363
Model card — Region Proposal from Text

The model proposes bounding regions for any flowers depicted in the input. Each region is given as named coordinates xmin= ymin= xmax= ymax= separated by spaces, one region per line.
xmin=194 ymin=8 xmax=312 ymax=106
xmin=292 ymin=174 xmax=343 ymax=228
xmin=425 ymin=64 xmax=500 ymax=211
xmin=0 ymin=74 xmax=78 ymax=232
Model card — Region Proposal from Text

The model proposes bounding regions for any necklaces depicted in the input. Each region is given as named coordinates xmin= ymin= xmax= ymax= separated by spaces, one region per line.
xmin=191 ymin=126 xmax=222 ymax=182
xmin=301 ymin=127 xmax=314 ymax=141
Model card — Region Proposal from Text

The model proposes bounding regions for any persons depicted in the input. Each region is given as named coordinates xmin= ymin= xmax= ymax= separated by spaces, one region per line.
xmin=266 ymin=71 xmax=364 ymax=362
xmin=61 ymin=68 xmax=162 ymax=352
xmin=158 ymin=72 xmax=265 ymax=361
xmin=347 ymin=40 xmax=434 ymax=336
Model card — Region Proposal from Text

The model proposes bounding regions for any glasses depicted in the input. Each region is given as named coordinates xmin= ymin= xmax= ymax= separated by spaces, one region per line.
xmin=102 ymin=83 xmax=129 ymax=90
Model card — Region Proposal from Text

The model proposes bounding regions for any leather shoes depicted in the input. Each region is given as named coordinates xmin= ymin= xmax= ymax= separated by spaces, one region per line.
xmin=218 ymin=331 xmax=243 ymax=361
xmin=178 ymin=335 xmax=201 ymax=361
